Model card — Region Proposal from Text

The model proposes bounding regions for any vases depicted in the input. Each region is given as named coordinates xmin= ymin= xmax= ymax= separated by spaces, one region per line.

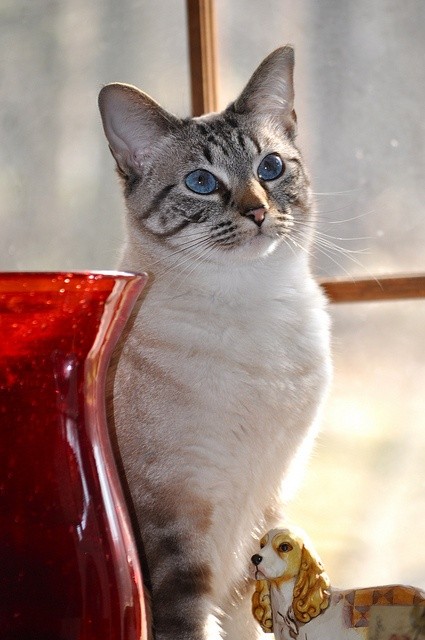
xmin=1 ymin=271 xmax=150 ymax=640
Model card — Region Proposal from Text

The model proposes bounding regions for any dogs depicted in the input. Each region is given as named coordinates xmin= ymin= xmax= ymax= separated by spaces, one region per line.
xmin=248 ymin=526 xmax=425 ymax=640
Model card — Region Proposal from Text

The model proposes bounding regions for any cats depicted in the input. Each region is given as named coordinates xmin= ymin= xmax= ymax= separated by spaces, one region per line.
xmin=97 ymin=46 xmax=334 ymax=639
